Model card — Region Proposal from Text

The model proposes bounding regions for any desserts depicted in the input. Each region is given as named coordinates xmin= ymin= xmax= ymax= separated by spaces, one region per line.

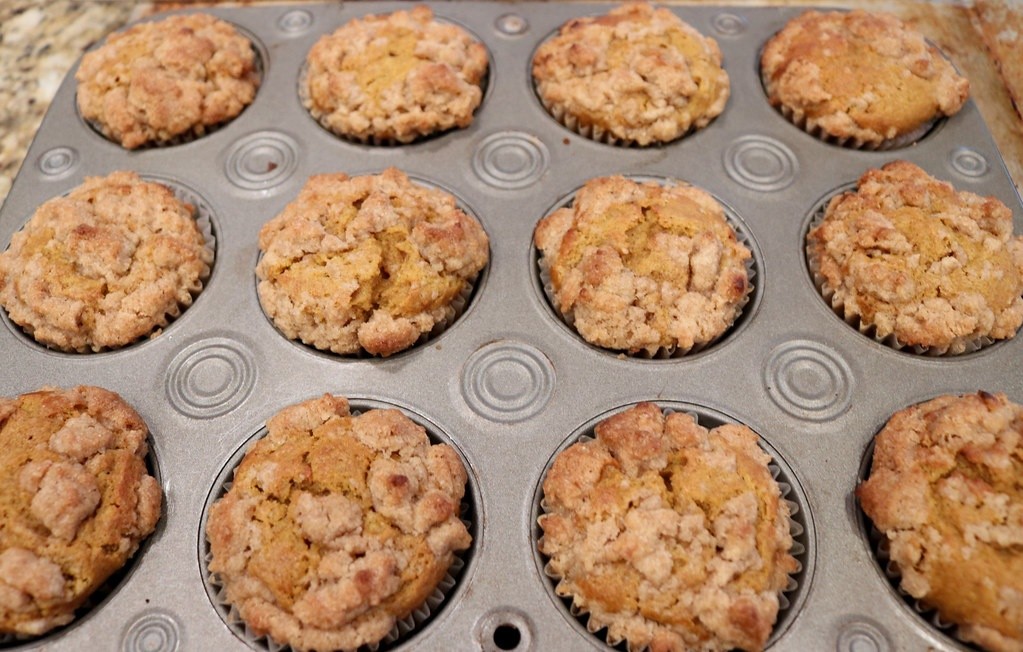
xmin=0 ymin=0 xmax=1023 ymax=652
